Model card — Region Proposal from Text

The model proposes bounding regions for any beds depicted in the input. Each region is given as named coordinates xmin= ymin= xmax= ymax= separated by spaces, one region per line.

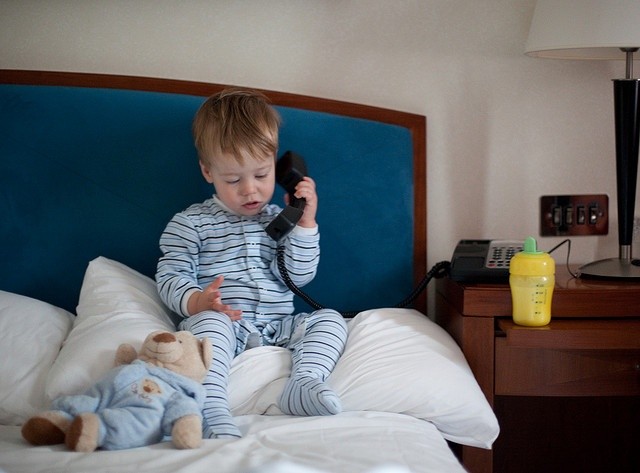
xmin=0 ymin=68 xmax=469 ymax=473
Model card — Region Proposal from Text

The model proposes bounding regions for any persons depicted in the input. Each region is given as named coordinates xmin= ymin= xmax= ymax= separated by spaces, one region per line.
xmin=157 ymin=88 xmax=347 ymax=441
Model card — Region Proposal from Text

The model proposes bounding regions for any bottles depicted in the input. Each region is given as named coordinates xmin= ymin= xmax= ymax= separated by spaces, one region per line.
xmin=509 ymin=251 xmax=557 ymax=327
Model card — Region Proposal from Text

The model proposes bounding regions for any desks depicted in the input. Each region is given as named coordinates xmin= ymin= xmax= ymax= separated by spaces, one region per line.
xmin=439 ymin=274 xmax=640 ymax=472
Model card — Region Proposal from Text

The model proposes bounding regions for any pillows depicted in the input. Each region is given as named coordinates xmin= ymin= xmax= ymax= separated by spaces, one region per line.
xmin=0 ymin=289 xmax=77 ymax=427
xmin=47 ymin=255 xmax=501 ymax=452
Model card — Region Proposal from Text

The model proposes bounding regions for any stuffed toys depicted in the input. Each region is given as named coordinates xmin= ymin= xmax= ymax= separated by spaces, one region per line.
xmin=23 ymin=331 xmax=212 ymax=453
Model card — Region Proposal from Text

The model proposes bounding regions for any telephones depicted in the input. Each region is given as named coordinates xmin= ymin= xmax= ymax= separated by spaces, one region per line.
xmin=266 ymin=152 xmax=524 ymax=318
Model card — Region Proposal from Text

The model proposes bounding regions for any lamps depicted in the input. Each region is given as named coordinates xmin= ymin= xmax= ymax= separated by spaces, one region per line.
xmin=522 ymin=0 xmax=640 ymax=283
